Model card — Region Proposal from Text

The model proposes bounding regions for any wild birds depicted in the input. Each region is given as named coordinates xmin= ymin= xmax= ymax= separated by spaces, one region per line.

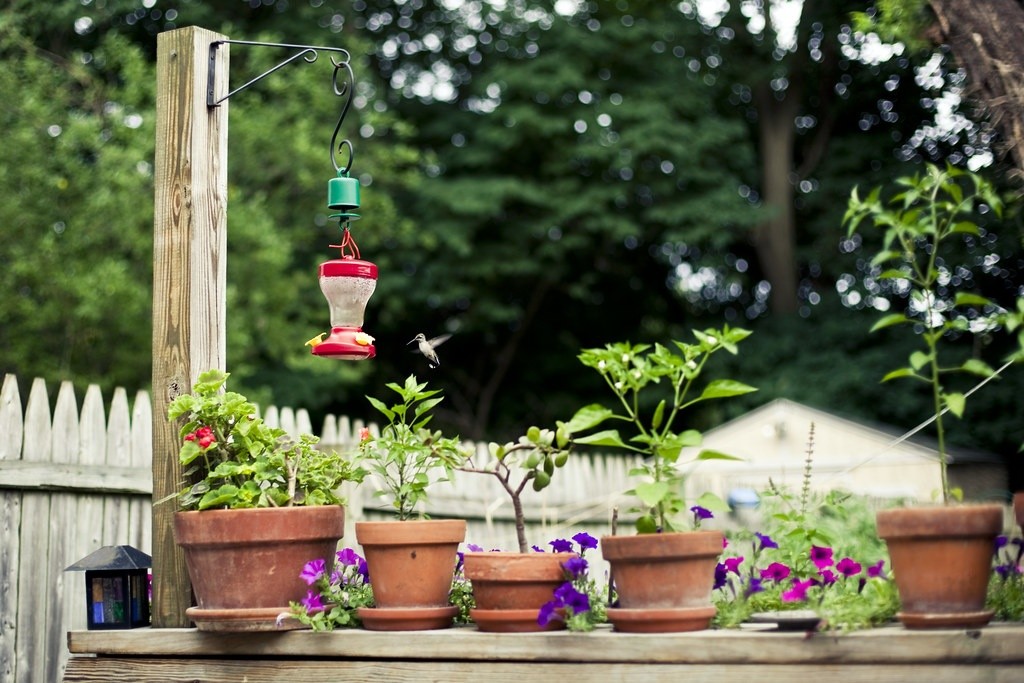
xmin=406 ymin=333 xmax=453 ymax=369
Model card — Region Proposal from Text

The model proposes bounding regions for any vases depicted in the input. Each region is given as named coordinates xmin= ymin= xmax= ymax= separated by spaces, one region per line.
xmin=174 ymin=506 xmax=345 ymax=632
xmin=356 ymin=520 xmax=466 ymax=630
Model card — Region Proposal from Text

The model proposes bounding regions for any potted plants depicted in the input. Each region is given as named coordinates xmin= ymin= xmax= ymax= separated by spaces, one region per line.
xmin=556 ymin=322 xmax=760 ymax=633
xmin=418 ymin=421 xmax=578 ymax=632
xmin=841 ymin=161 xmax=1024 ymax=628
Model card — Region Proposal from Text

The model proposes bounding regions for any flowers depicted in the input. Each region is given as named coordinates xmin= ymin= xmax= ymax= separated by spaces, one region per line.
xmin=152 ymin=368 xmax=370 ymax=511
xmin=350 ymin=375 xmax=455 ymax=522
